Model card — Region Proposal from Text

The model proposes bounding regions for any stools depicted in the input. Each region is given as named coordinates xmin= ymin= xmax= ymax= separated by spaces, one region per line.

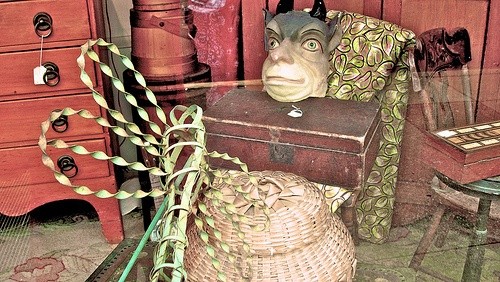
xmin=407 ymin=169 xmax=500 ymax=282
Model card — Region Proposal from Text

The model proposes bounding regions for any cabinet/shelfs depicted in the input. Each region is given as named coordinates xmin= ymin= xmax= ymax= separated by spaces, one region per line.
xmin=0 ymin=0 xmax=126 ymax=248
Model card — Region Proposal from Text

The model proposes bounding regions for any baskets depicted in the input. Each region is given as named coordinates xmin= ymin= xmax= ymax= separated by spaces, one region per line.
xmin=178 ymin=169 xmax=356 ymax=282
